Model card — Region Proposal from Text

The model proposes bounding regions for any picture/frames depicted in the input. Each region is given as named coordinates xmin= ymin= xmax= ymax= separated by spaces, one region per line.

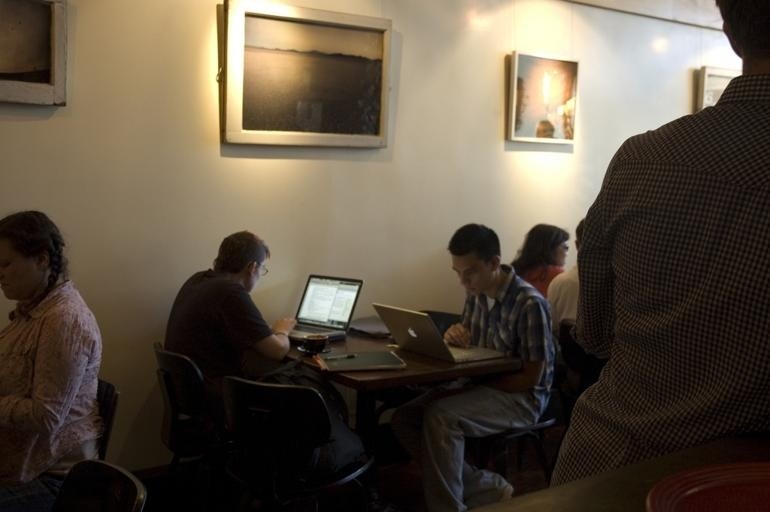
xmin=1 ymin=0 xmax=67 ymax=109
xmin=693 ymin=65 xmax=741 ymax=116
xmin=218 ymin=0 xmax=395 ymax=152
xmin=508 ymin=51 xmax=582 ymax=149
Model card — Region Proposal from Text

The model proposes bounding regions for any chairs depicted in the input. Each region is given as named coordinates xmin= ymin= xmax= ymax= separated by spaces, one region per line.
xmin=414 ymin=305 xmax=591 ymax=508
xmin=152 ymin=337 xmax=369 ymax=509
xmin=49 ymin=378 xmax=148 ymax=511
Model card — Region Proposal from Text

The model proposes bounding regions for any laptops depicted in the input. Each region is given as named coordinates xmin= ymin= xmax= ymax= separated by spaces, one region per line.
xmin=372 ymin=301 xmax=506 ymax=365
xmin=287 ymin=273 xmax=364 ymax=346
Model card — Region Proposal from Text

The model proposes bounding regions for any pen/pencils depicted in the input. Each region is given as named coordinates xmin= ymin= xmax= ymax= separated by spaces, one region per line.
xmin=327 ymin=354 xmax=355 ymax=360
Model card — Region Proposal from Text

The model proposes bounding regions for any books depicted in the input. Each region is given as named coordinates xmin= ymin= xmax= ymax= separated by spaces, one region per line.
xmin=312 ymin=350 xmax=407 ymax=372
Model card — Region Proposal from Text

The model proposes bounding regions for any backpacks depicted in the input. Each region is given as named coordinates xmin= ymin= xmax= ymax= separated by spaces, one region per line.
xmin=261 ymin=362 xmax=349 ymax=426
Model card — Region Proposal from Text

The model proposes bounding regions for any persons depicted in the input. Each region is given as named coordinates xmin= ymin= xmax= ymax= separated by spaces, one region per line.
xmin=511 ymin=224 xmax=569 ymax=297
xmin=165 ymin=232 xmax=367 ymax=472
xmin=391 ymin=221 xmax=555 ymax=512
xmin=1 ymin=209 xmax=104 ymax=507
xmin=549 ymin=0 xmax=770 ymax=489
xmin=546 ymin=218 xmax=588 ymax=340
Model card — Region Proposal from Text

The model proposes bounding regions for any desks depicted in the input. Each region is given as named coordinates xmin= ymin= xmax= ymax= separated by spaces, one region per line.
xmin=265 ymin=316 xmax=523 ymax=510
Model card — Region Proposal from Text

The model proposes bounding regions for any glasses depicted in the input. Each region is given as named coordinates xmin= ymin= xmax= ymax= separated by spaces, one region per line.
xmin=247 ymin=261 xmax=268 ymax=277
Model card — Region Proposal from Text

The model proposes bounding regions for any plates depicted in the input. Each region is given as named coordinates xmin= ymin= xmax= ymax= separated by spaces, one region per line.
xmin=298 ymin=345 xmax=330 ymax=352
xmin=646 ymin=461 xmax=769 ymax=512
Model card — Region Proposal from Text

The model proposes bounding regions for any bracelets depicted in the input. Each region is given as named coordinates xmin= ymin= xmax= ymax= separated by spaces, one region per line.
xmin=272 ymin=330 xmax=288 ymax=336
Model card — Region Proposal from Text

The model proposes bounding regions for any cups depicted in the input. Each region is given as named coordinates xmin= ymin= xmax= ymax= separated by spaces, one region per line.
xmin=305 ymin=335 xmax=328 ymax=351
